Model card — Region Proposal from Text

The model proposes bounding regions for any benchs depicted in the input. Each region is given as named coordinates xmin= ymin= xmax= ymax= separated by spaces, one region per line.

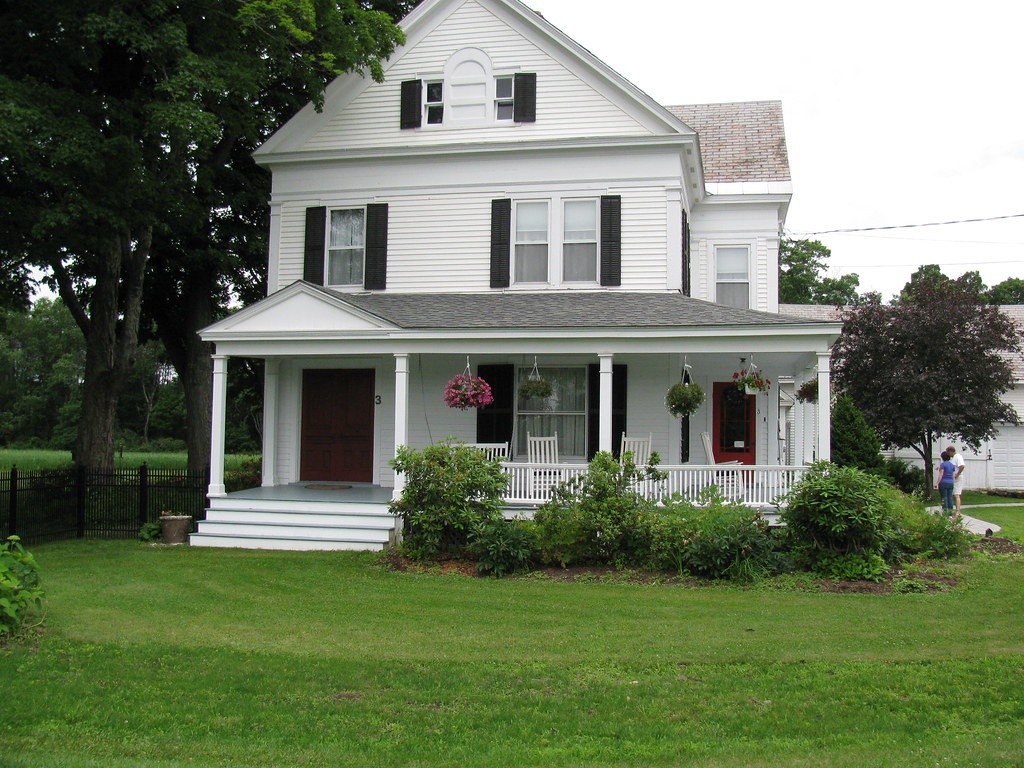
xmin=437 ymin=442 xmax=510 ymax=499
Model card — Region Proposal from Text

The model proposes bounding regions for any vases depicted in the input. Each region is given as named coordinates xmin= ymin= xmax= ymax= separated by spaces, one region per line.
xmin=805 ymin=395 xmax=820 ymax=406
xmin=744 ymin=383 xmax=759 ymax=395
xmin=161 ymin=515 xmax=192 ymax=543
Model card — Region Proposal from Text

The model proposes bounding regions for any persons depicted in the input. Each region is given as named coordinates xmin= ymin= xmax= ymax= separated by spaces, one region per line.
xmin=937 ymin=446 xmax=965 ymax=520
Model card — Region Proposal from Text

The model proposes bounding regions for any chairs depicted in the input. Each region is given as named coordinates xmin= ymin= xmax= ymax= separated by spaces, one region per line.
xmin=525 ymin=431 xmax=568 ymax=496
xmin=619 ymin=432 xmax=655 ymax=498
xmin=701 ymin=432 xmax=746 ymax=498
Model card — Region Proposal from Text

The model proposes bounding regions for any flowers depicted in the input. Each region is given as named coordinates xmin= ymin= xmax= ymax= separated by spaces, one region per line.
xmin=159 ymin=509 xmax=193 ymax=518
xmin=663 ymin=383 xmax=707 ymax=420
xmin=443 ymin=373 xmax=493 ymax=412
xmin=732 ymin=369 xmax=773 ymax=396
xmin=796 ymin=376 xmax=820 ymax=407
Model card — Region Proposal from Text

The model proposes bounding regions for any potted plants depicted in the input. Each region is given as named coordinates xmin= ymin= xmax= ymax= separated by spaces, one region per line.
xmin=521 ymin=380 xmax=559 ymax=400
